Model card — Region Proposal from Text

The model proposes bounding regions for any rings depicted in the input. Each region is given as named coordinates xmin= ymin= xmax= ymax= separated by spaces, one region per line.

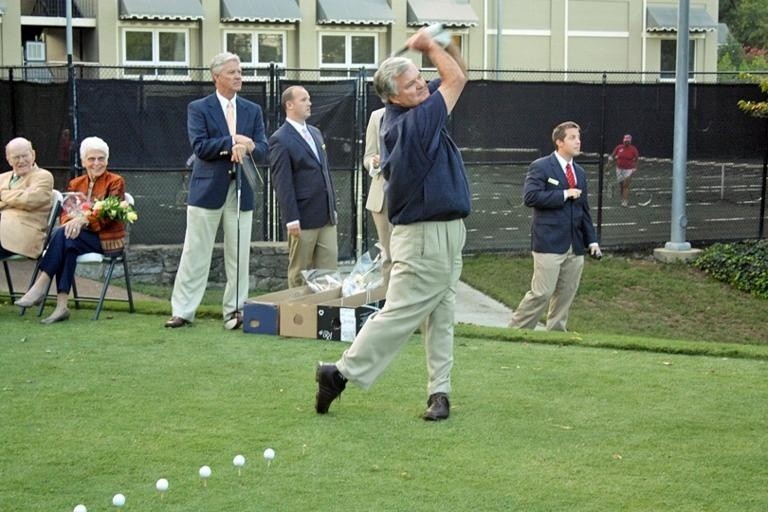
xmin=73 ymin=228 xmax=77 ymax=233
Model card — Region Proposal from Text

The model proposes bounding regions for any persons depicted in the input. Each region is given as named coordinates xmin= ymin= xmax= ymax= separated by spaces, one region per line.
xmin=314 ymin=25 xmax=473 ymax=421
xmin=0 ymin=136 xmax=55 ymax=261
xmin=13 ymin=134 xmax=126 ymax=325
xmin=606 ymin=133 xmax=639 ymax=206
xmin=363 ymin=105 xmax=395 ymax=293
xmin=268 ymin=84 xmax=340 ymax=288
xmin=58 ymin=129 xmax=71 ymax=163
xmin=504 ymin=120 xmax=602 ymax=331
xmin=165 ymin=52 xmax=270 ymax=329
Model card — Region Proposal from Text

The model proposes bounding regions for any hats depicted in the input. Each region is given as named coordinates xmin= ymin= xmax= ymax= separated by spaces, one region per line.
xmin=623 ymin=135 xmax=632 ymax=142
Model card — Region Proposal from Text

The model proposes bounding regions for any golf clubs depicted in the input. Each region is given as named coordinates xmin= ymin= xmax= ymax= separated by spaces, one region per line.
xmin=224 ymin=162 xmax=241 ymax=330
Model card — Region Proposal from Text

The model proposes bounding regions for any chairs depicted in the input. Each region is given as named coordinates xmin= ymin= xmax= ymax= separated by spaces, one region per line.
xmin=3 ymin=187 xmax=63 ymax=316
xmin=39 ymin=192 xmax=135 ymax=320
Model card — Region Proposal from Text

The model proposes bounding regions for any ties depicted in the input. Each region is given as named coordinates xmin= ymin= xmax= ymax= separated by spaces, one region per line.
xmin=226 ymin=102 xmax=235 ymax=137
xmin=566 ymin=164 xmax=576 ymax=189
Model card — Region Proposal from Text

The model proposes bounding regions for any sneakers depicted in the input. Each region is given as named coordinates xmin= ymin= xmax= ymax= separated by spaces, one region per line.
xmin=621 ymin=199 xmax=629 ymax=206
xmin=315 ymin=360 xmax=347 ymax=414
xmin=164 ymin=316 xmax=186 ymax=327
xmin=422 ymin=396 xmax=450 ymax=421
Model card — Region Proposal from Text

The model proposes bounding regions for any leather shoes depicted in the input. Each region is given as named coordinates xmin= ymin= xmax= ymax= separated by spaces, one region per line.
xmin=15 ymin=294 xmax=44 ymax=308
xmin=41 ymin=309 xmax=70 ymax=324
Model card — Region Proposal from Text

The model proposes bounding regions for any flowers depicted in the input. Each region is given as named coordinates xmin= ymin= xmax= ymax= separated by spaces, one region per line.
xmin=85 ymin=194 xmax=138 ymax=231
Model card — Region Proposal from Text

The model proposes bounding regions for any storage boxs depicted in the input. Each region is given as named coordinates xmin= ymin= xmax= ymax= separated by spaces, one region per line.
xmin=241 ymin=283 xmax=387 ymax=342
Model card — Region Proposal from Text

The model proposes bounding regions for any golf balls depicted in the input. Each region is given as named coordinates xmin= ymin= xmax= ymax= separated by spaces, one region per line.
xmin=74 ymin=448 xmax=275 ymax=512
